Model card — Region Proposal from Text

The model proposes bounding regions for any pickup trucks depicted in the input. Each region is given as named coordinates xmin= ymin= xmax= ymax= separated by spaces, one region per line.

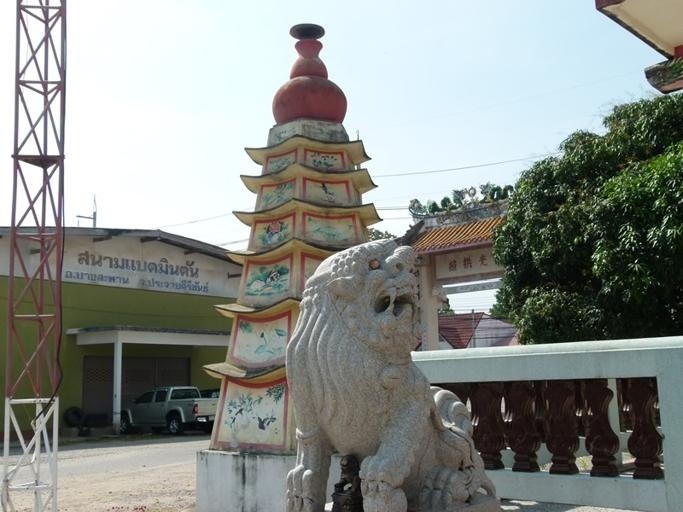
xmin=119 ymin=385 xmax=220 ymax=438
